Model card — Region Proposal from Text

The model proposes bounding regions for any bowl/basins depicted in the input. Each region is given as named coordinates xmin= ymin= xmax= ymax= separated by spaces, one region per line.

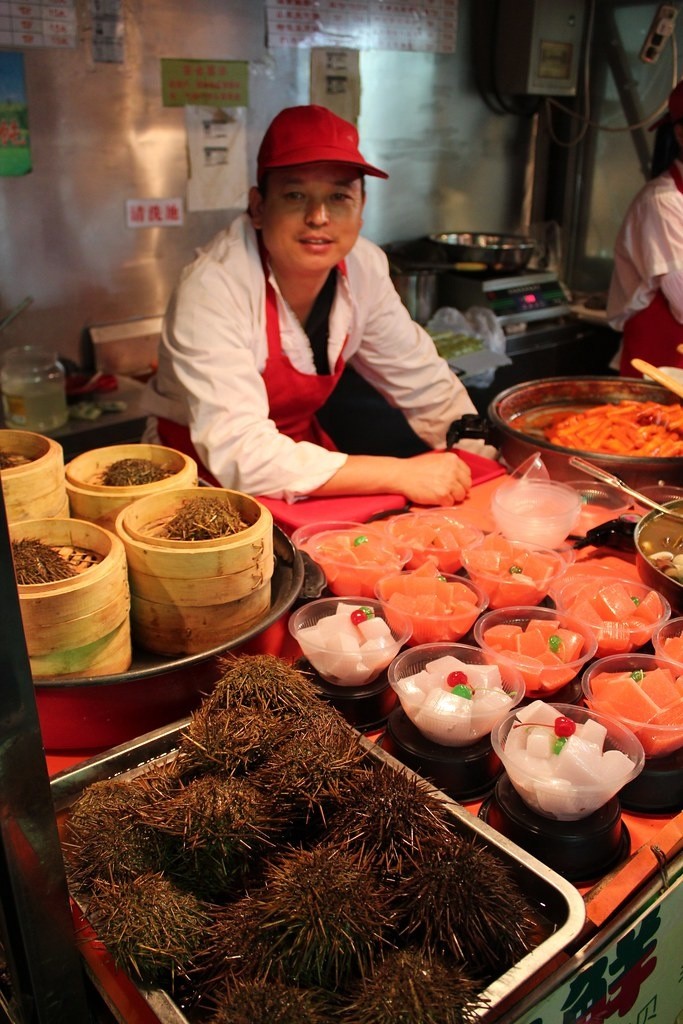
xmin=474 ymin=603 xmax=598 ymax=699
xmin=653 ymin=616 xmax=683 ymax=664
xmin=557 ymin=580 xmax=673 ymax=656
xmin=412 ymin=507 xmax=501 ymax=540
xmin=292 ymin=522 xmax=377 ymax=555
xmin=389 ymin=640 xmax=527 ymax=749
xmin=493 ymin=702 xmax=646 ymax=822
xmin=550 ymin=563 xmax=627 ymax=600
xmin=373 ymin=571 xmax=490 ymax=648
xmin=582 ymin=653 xmax=683 ymax=759
xmin=310 ymin=531 xmax=412 ymax=594
xmin=630 ymin=486 xmax=683 ymax=511
xmin=289 ymin=596 xmax=413 ymax=686
xmin=385 ymin=514 xmax=481 ymax=576
xmin=462 ymin=540 xmax=565 ymax=612
xmin=424 ymin=232 xmax=533 ymax=275
xmin=492 ymin=452 xmax=630 ymax=551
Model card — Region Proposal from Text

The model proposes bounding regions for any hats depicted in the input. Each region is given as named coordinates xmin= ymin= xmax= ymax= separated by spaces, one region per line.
xmin=648 ymin=80 xmax=683 ymax=133
xmin=257 ymin=104 xmax=390 ymax=180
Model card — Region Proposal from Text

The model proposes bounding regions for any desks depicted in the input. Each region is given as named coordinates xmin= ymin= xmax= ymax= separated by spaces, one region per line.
xmin=39 ymin=372 xmax=149 ymax=468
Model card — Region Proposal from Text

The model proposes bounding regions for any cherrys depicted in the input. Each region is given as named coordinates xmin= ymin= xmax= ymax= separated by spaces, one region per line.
xmin=447 ymin=671 xmax=467 ymax=687
xmin=360 ymin=607 xmax=373 ymax=618
xmin=514 ymin=716 xmax=576 ymax=737
xmin=452 ymin=685 xmax=507 ymax=699
xmin=352 ymin=611 xmax=382 ymax=625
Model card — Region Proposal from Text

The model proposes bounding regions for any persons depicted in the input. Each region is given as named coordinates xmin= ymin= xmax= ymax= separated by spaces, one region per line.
xmin=138 ymin=105 xmax=478 ymax=505
xmin=606 ymin=80 xmax=683 ymax=379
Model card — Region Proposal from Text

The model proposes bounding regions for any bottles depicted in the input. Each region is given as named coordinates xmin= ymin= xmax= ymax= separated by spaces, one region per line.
xmin=0 ymin=345 xmax=68 ymax=431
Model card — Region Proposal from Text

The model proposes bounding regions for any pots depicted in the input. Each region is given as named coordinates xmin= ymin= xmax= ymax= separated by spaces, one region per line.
xmin=633 ymin=498 xmax=683 ymax=614
